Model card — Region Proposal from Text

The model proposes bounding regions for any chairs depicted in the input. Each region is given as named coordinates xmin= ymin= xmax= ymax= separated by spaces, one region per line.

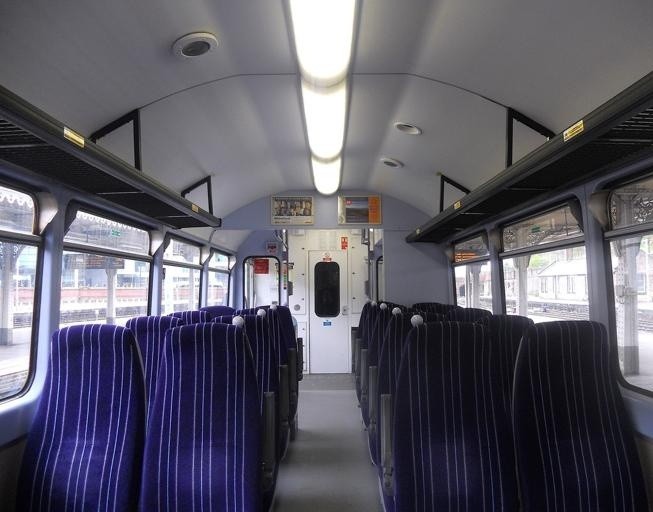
xmin=441 ymin=308 xmax=494 ymax=324
xmin=391 ymin=323 xmax=512 ymax=511
xmin=248 ymin=306 xmax=298 ymax=383
xmin=511 ymin=320 xmax=651 ymax=512
xmin=124 ymin=316 xmax=188 ymax=424
xmin=412 ymin=302 xmax=464 ymax=313
xmin=209 ymin=315 xmax=290 ymax=464
xmin=232 ymin=307 xmax=298 ymax=425
xmin=370 ymin=312 xmax=451 ymax=498
xmin=464 ymin=314 xmax=535 ymax=411
xmin=362 ymin=305 xmax=414 ymax=431
xmin=166 ymin=311 xmax=211 ymax=324
xmin=197 ymin=306 xmax=235 ymax=315
xmin=12 ymin=323 xmax=147 ymax=512
xmin=355 ymin=301 xmax=408 ymax=402
xmin=141 ymin=323 xmax=275 ymax=512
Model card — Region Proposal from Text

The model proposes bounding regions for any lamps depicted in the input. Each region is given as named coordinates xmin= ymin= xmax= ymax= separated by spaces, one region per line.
xmin=311 ymin=153 xmax=340 ymax=195
xmin=288 ymin=0 xmax=358 ymax=86
xmin=298 ymin=77 xmax=348 ymax=158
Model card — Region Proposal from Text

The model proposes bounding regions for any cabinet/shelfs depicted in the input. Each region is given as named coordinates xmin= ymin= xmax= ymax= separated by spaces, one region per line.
xmin=403 ymin=105 xmax=652 ymax=245
xmin=0 ymin=108 xmax=223 ymax=230
